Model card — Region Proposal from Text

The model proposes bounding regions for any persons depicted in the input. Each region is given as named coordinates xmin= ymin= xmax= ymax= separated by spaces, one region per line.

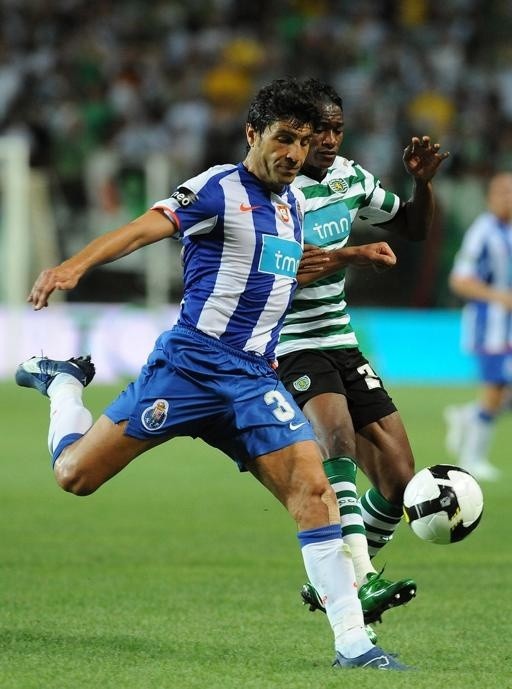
xmin=13 ymin=79 xmax=411 ymax=671
xmin=245 ymin=83 xmax=454 ymax=627
xmin=437 ymin=172 xmax=510 ymax=478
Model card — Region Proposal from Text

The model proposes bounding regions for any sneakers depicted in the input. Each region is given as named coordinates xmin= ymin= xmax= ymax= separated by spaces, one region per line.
xmin=14 ymin=352 xmax=98 ymax=396
xmin=447 ymin=406 xmax=498 ymax=484
xmin=330 ymin=643 xmax=420 ymax=670
xmin=300 ymin=570 xmax=417 ymax=624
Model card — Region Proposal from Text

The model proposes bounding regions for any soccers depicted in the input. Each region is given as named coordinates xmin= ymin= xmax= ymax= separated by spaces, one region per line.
xmin=402 ymin=463 xmax=483 ymax=545
